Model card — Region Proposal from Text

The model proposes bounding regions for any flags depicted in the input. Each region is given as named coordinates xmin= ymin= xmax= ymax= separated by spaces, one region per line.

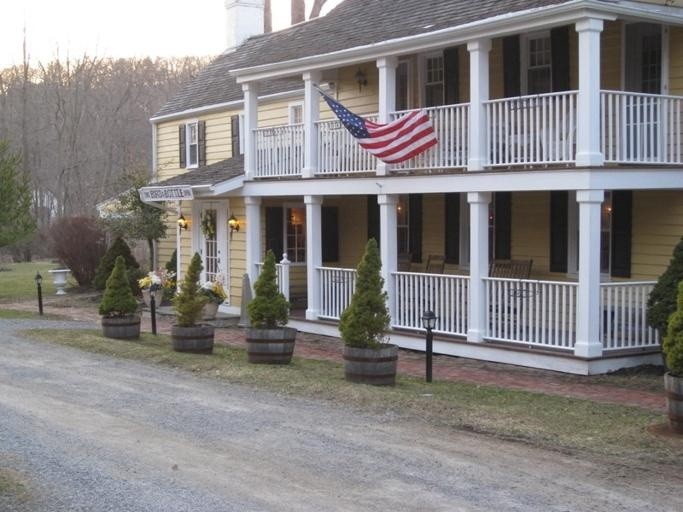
xmin=311 ymin=77 xmax=438 ymax=166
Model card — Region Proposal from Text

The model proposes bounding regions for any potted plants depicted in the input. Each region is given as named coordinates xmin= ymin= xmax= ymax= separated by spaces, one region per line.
xmin=200 ymin=280 xmax=228 ymax=320
xmin=337 ymin=238 xmax=399 ymax=387
xmin=97 ymin=254 xmax=141 ymax=339
xmin=243 ymin=248 xmax=297 ymax=364
xmin=660 ymin=282 xmax=682 ymax=435
xmin=137 ymin=270 xmax=171 ymax=309
xmin=169 ymin=251 xmax=215 ymax=354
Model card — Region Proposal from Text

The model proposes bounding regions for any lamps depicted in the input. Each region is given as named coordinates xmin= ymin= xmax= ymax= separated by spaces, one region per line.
xmin=226 ymin=211 xmax=239 ymax=240
xmin=177 ymin=212 xmax=187 ymax=235
xmin=420 ymin=311 xmax=438 ymax=382
xmin=34 ymin=269 xmax=42 ymax=315
xmin=354 ymin=67 xmax=367 ymax=93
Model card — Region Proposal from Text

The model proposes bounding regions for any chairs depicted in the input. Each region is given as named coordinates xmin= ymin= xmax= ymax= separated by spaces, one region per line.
xmin=397 ymin=252 xmax=533 ymax=323
